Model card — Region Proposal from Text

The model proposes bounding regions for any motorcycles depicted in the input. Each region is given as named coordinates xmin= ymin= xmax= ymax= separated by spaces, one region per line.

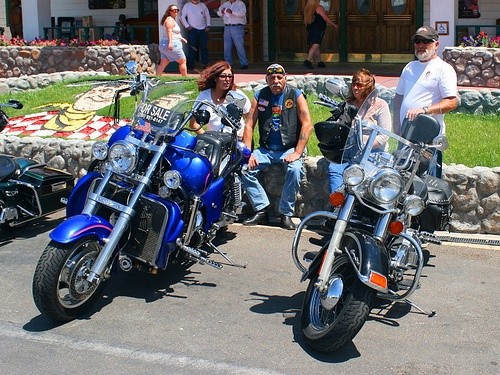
xmin=32 ymin=60 xmax=250 ymax=320
xmin=293 ymin=79 xmax=453 ymax=354
xmin=0 ymin=99 xmax=74 ymax=227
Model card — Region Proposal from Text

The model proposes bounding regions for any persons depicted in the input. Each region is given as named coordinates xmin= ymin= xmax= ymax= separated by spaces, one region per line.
xmin=179 ymin=0 xmax=211 ymax=71
xmin=302 ymin=0 xmax=338 ymax=69
xmin=111 ymin=13 xmax=135 ymax=45
xmin=392 ymin=25 xmax=459 ymax=179
xmin=216 ymin=0 xmax=250 ymax=69
xmin=189 ymin=60 xmax=253 ymax=178
xmin=240 ymin=64 xmax=314 ymax=231
xmin=155 ymin=4 xmax=188 ymax=76
xmin=328 ymin=68 xmax=392 ymax=214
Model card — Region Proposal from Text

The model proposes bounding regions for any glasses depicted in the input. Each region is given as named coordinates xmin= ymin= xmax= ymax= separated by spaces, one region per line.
xmin=413 ymin=38 xmax=436 ymax=44
xmin=170 ymin=8 xmax=180 ymax=11
xmin=217 ymin=74 xmax=234 ymax=79
xmin=267 ymin=67 xmax=285 ymax=74
xmin=351 ymin=81 xmax=372 ymax=87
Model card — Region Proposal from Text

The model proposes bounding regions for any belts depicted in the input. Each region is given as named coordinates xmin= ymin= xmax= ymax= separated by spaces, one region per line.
xmin=224 ymin=24 xmax=244 ymax=26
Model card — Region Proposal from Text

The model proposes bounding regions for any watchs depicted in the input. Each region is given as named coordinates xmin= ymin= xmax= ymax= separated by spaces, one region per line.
xmin=423 ymin=105 xmax=429 ymax=114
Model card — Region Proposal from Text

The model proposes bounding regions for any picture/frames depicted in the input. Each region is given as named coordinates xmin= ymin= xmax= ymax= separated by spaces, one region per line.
xmin=434 ymin=20 xmax=450 ymax=35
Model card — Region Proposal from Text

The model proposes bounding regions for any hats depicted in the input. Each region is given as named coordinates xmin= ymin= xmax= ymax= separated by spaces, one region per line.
xmin=412 ymin=25 xmax=439 ymax=40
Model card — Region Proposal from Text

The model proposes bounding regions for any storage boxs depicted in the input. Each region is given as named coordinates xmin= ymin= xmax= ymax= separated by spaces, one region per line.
xmin=79 ymin=28 xmax=104 ymax=42
xmin=81 ymin=16 xmax=93 ymax=26
xmin=47 ymin=29 xmax=56 ymax=39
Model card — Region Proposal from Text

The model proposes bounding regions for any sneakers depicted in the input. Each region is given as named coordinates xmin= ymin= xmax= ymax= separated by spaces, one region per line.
xmin=302 ymin=60 xmax=314 ymax=69
xmin=318 ymin=61 xmax=326 ymax=67
xmin=240 ymin=65 xmax=248 ymax=69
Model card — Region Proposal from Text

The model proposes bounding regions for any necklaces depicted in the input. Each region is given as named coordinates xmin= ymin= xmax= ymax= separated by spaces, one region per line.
xmin=213 ymin=89 xmax=225 ymax=102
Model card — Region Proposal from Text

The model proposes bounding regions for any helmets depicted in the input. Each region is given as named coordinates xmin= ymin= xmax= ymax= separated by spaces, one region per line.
xmin=313 ymin=101 xmax=358 ymax=163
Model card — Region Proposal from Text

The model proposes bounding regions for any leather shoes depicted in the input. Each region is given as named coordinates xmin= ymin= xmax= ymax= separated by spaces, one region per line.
xmin=242 ymin=211 xmax=269 ymax=226
xmin=280 ymin=214 xmax=296 ymax=229
